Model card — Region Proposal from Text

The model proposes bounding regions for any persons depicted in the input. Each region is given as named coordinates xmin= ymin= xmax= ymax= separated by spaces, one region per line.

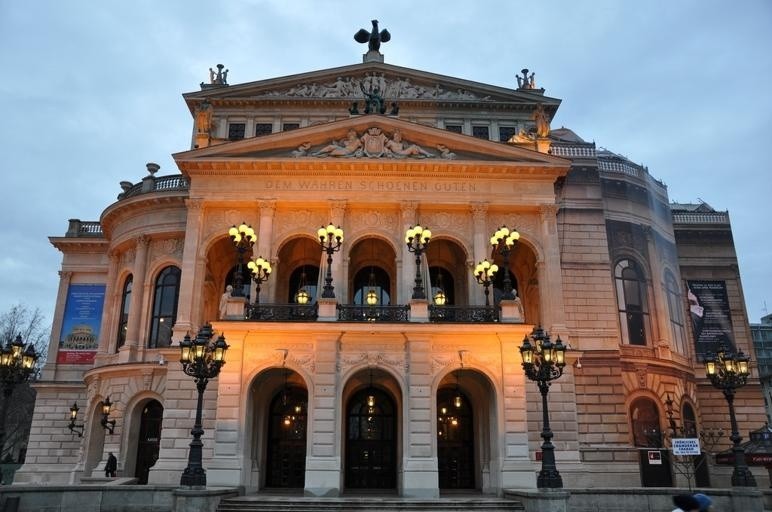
xmin=311 ymin=130 xmax=364 ymax=158
xmin=101 ymin=451 xmax=118 ymax=476
xmin=195 ymin=97 xmax=214 ymax=132
xmin=251 ymin=71 xmax=490 ymax=102
xmin=670 ymin=494 xmax=699 ymax=512
xmin=529 ymin=102 xmax=550 ymax=138
xmin=386 ymin=131 xmax=437 ymax=159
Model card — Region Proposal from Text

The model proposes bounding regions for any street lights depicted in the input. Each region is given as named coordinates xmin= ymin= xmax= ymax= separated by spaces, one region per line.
xmin=229 ymin=221 xmax=272 ymax=319
xmin=405 ymin=223 xmax=432 ymax=299
xmin=179 ymin=321 xmax=230 ymax=485
xmin=518 ymin=322 xmax=566 ymax=488
xmin=0 ymin=332 xmax=36 ymax=482
xmin=474 ymin=224 xmax=520 ymax=321
xmin=316 ymin=222 xmax=344 ymax=298
xmin=703 ymin=347 xmax=756 ymax=486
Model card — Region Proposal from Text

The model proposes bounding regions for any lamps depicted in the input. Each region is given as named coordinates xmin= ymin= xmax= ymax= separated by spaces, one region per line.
xmin=367 ymin=368 xmax=378 ymax=420
xmin=70 ymin=396 xmax=117 ymax=437
xmin=281 ymin=369 xmax=293 ymax=429
xmin=365 ymin=239 xmax=380 ymax=305
xmin=434 ymin=239 xmax=448 ymax=305
xmin=296 ymin=238 xmax=310 ymax=305
xmin=454 ymin=369 xmax=464 ymax=411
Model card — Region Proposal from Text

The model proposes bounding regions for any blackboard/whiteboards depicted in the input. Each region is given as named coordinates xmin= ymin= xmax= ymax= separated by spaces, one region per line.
xmin=672 ymin=438 xmax=701 ymax=455
